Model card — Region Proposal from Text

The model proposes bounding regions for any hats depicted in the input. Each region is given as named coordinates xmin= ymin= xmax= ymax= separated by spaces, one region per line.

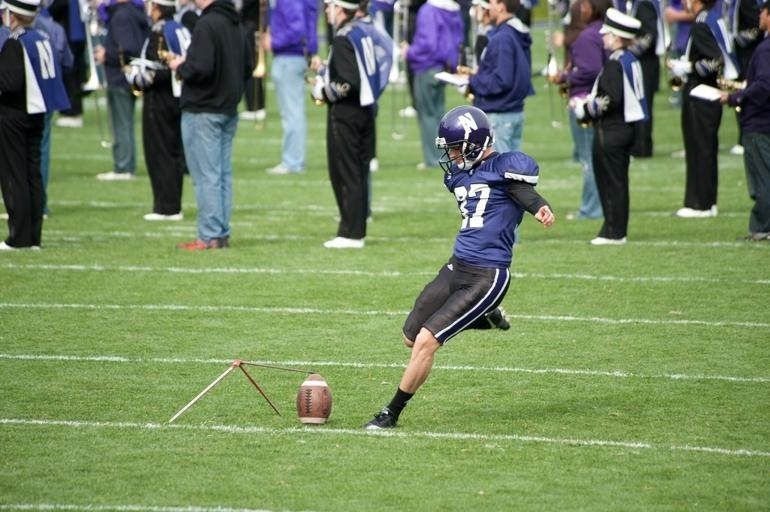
xmin=1 ymin=0 xmax=41 ymax=17
xmin=323 ymin=0 xmax=359 ymax=10
xmin=152 ymin=1 xmax=176 ymax=6
xmin=599 ymin=8 xmax=642 ymax=40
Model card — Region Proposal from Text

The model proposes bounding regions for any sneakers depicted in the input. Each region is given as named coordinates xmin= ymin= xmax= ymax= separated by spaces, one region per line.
xmin=1 ymin=241 xmax=41 ymax=254
xmin=325 ymin=236 xmax=365 ymax=250
xmin=488 ymin=307 xmax=510 ymax=330
xmin=738 ymin=229 xmax=769 ymax=246
xmin=96 ymin=170 xmax=135 ymax=182
xmin=175 ymin=237 xmax=230 ymax=253
xmin=676 ymin=206 xmax=719 ymax=220
xmin=591 ymin=237 xmax=628 ymax=245
xmin=54 ymin=116 xmax=83 ymax=130
xmin=266 ymin=164 xmax=301 ymax=176
xmin=144 ymin=212 xmax=183 ymax=223
xmin=238 ymin=109 xmax=266 ymax=121
xmin=358 ymin=406 xmax=400 ymax=430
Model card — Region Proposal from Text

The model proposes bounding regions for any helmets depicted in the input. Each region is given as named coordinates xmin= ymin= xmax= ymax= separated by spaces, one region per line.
xmin=435 ymin=104 xmax=496 ymax=176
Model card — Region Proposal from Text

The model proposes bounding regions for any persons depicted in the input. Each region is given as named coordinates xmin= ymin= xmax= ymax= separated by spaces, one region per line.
xmin=361 ymin=104 xmax=556 ymax=428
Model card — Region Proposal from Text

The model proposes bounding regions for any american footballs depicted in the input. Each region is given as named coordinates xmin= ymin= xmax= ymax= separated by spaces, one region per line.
xmin=297 ymin=374 xmax=332 ymax=424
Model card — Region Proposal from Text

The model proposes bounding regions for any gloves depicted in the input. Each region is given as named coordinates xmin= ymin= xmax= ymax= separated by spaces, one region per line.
xmin=670 ymin=60 xmax=691 ymax=76
xmin=575 ymin=98 xmax=586 ymax=120
xmin=313 ymin=76 xmax=323 ymax=100
xmin=124 ymin=67 xmax=139 ymax=84
